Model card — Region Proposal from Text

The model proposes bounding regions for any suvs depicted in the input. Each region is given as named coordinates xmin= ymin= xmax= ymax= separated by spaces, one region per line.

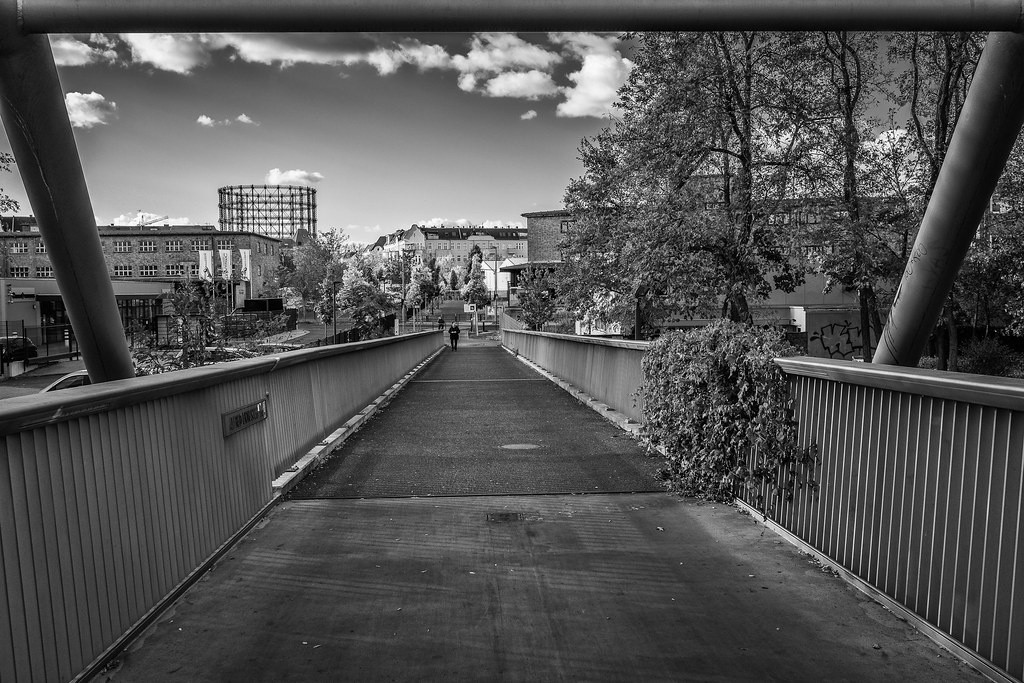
xmin=176 ymin=347 xmax=256 ymax=366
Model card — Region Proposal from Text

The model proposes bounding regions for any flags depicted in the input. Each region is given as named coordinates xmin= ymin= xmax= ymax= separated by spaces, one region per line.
xmin=218 ymin=249 xmax=234 ymax=280
xmin=238 ymin=249 xmax=250 ymax=281
xmin=197 ymin=249 xmax=214 ymax=283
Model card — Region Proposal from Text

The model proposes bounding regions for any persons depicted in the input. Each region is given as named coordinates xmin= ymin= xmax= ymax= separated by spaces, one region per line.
xmin=437 ymin=317 xmax=446 ymax=329
xmin=448 ymin=322 xmax=461 ymax=352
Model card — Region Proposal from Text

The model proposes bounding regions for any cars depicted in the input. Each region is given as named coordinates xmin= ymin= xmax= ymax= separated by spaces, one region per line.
xmin=132 ymin=353 xmax=178 ymax=376
xmin=38 ymin=368 xmax=91 ymax=393
xmin=0 ymin=336 xmax=38 ymax=364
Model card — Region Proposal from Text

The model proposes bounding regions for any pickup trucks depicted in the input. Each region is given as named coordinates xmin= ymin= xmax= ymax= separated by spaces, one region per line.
xmin=220 ymin=307 xmax=271 ymax=325
